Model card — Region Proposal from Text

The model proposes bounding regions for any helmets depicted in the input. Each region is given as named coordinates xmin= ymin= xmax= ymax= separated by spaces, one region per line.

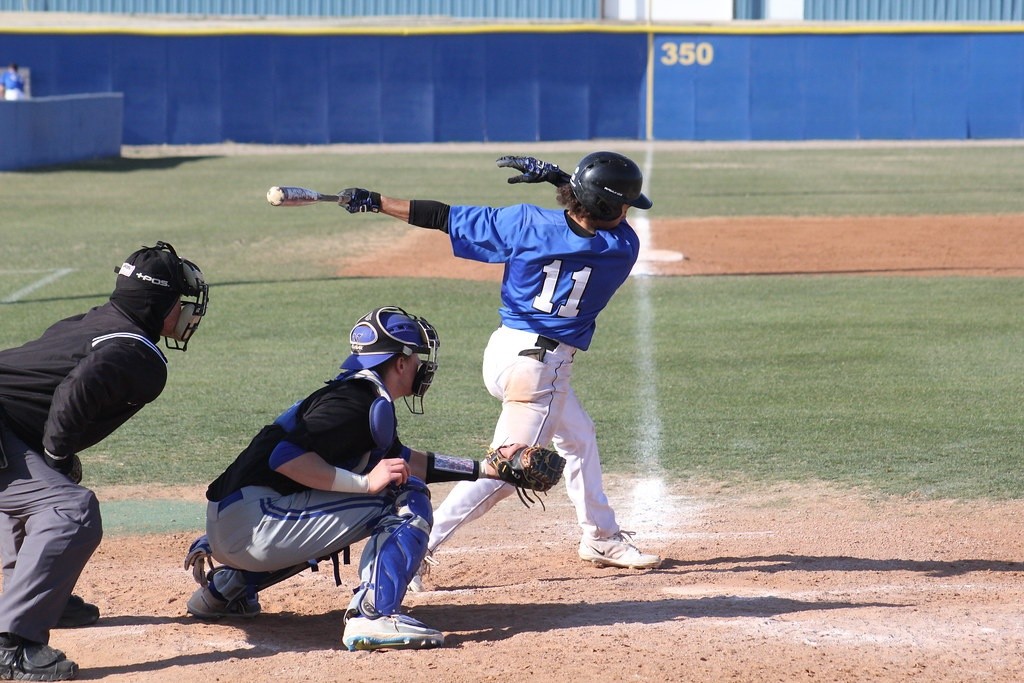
xmin=341 ymin=313 xmax=423 ymax=371
xmin=569 ymin=151 xmax=652 ymax=221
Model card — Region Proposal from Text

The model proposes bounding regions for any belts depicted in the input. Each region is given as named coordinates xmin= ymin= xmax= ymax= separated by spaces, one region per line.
xmin=499 ymin=320 xmax=560 ymax=353
xmin=217 ymin=490 xmax=244 ymax=512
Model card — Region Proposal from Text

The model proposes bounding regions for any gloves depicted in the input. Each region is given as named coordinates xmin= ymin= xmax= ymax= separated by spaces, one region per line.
xmin=336 ymin=187 xmax=382 ymax=214
xmin=184 ymin=535 xmax=215 ymax=587
xmin=44 ymin=448 xmax=82 ymax=484
xmin=496 ymin=154 xmax=561 ymax=186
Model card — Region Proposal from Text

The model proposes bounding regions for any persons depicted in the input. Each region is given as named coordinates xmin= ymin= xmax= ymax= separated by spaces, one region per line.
xmin=338 ymin=151 xmax=661 ymax=592
xmin=184 ymin=305 xmax=528 ymax=648
xmin=0 ymin=63 xmax=26 ymax=100
xmin=0 ymin=242 xmax=209 ymax=683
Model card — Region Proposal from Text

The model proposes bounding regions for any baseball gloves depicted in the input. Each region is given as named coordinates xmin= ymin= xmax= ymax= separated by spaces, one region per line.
xmin=483 ymin=437 xmax=568 ymax=512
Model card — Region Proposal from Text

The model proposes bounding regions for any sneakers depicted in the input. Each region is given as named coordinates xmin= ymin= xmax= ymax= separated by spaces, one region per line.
xmin=187 ymin=580 xmax=261 ymax=620
xmin=577 ymin=530 xmax=661 ymax=567
xmin=0 ymin=635 xmax=78 ymax=681
xmin=51 ymin=596 xmax=100 ymax=626
xmin=405 ymin=549 xmax=440 ymax=592
xmin=342 ymin=608 xmax=444 ymax=652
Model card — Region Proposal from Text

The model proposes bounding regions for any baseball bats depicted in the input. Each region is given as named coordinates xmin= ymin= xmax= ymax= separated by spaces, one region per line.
xmin=266 ymin=184 xmax=352 ymax=208
